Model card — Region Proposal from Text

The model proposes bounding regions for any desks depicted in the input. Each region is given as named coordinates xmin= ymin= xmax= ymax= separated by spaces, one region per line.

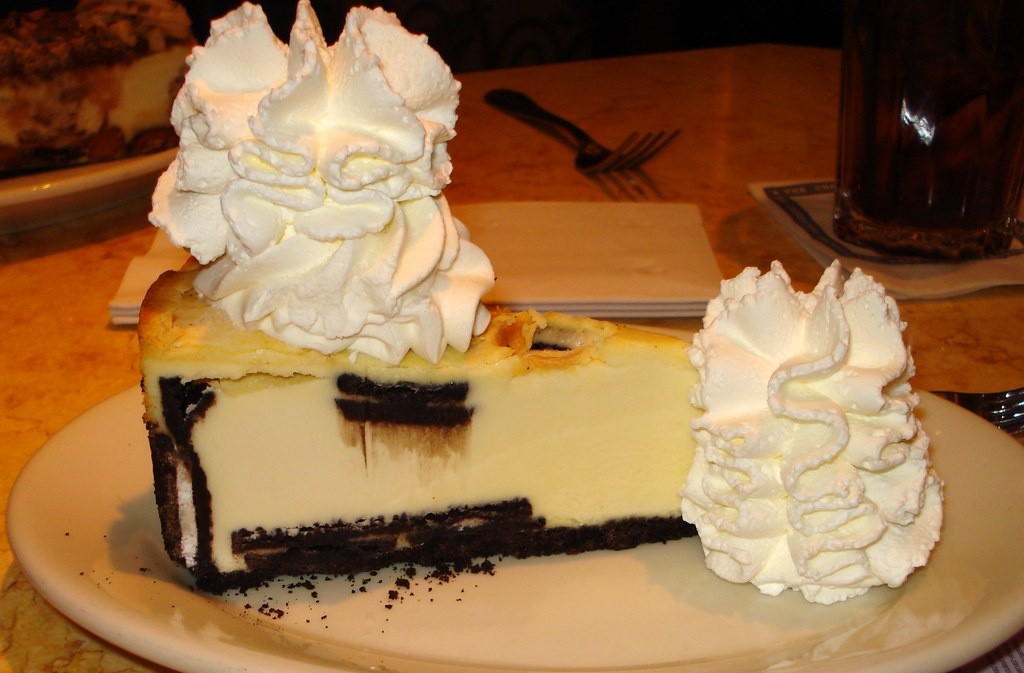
xmin=0 ymin=45 xmax=1024 ymax=673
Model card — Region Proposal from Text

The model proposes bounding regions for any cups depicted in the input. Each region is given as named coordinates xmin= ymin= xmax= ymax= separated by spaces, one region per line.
xmin=832 ymin=0 xmax=1024 ymax=262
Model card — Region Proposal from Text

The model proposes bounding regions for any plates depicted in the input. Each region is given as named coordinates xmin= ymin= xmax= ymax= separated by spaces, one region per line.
xmin=0 ymin=147 xmax=179 ymax=232
xmin=7 ymin=322 xmax=1024 ymax=672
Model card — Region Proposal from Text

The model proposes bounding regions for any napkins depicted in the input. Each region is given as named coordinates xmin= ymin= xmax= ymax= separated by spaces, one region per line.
xmin=449 ymin=202 xmax=723 ymax=318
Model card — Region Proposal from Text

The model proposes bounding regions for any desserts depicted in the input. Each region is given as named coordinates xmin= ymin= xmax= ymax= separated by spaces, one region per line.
xmin=138 ymin=0 xmax=946 ymax=603
xmin=0 ymin=0 xmax=200 ymax=178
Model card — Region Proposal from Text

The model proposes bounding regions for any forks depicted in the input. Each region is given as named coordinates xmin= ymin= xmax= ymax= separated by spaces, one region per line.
xmin=486 ymin=89 xmax=680 ymax=176
xmin=930 ymin=387 xmax=1024 ymax=436
